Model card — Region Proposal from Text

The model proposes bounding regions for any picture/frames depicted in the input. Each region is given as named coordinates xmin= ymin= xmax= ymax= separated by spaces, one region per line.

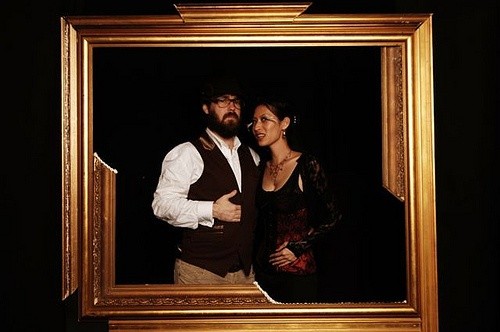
xmin=59 ymin=0 xmax=439 ymax=332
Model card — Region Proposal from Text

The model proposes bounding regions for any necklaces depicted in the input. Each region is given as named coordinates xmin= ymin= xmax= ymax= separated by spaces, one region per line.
xmin=268 ymin=150 xmax=293 ymax=184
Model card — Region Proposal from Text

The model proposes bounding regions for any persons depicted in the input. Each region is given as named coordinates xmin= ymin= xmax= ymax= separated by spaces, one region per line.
xmin=151 ymin=92 xmax=263 ymax=285
xmin=251 ymin=98 xmax=344 ymax=302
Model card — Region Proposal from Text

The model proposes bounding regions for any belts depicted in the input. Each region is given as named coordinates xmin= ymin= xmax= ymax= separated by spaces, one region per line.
xmin=175 ymin=251 xmax=241 ymax=273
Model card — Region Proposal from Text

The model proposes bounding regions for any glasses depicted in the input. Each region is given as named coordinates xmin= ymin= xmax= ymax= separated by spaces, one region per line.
xmin=212 ymin=98 xmax=245 ymax=109
